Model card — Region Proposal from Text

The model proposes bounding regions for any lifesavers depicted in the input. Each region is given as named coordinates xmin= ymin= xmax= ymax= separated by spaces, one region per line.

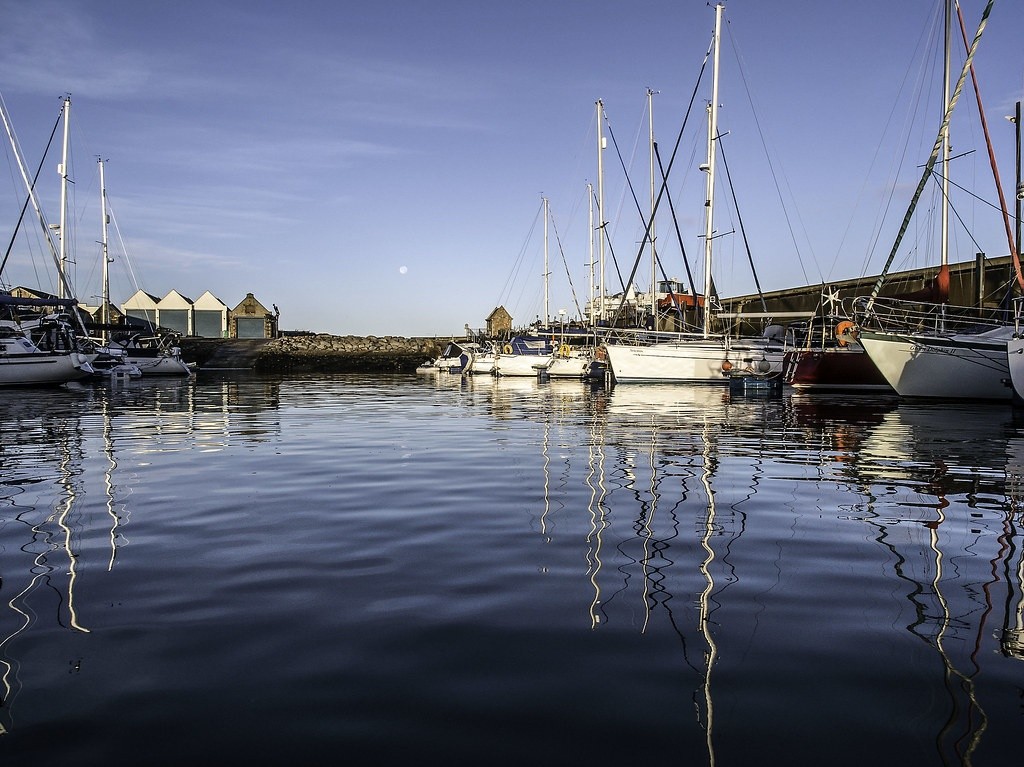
xmin=560 ymin=343 xmax=570 ymax=357
xmin=504 ymin=344 xmax=514 ymax=354
xmin=835 ymin=320 xmax=858 ymax=347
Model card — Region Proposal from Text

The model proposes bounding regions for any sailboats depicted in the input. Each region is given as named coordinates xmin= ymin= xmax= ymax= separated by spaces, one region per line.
xmin=0 ymin=90 xmax=197 ymax=382
xmin=416 ymin=0 xmax=1024 ymax=400
